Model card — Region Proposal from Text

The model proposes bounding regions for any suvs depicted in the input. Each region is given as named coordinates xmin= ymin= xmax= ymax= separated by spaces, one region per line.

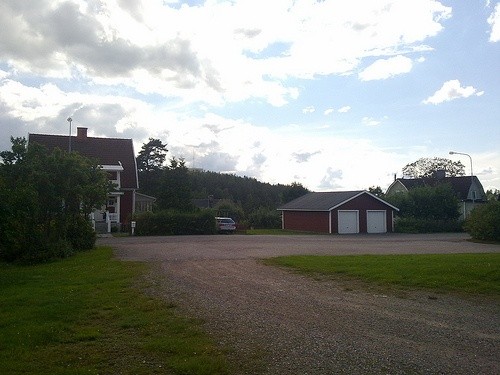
xmin=214 ymin=217 xmax=236 ymax=234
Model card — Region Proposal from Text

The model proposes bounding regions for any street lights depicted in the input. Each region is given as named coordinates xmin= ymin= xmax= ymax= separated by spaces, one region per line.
xmin=448 ymin=152 xmax=475 ymax=209
xmin=208 ymin=194 xmax=214 ymax=208
xmin=67 ymin=118 xmax=73 ymax=153
